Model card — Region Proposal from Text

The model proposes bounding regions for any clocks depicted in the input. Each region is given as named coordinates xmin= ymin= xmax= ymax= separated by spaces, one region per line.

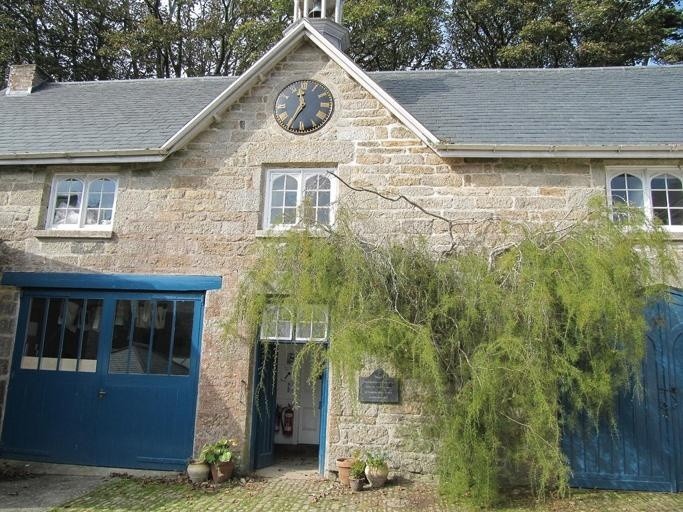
xmin=273 ymin=79 xmax=334 ymax=135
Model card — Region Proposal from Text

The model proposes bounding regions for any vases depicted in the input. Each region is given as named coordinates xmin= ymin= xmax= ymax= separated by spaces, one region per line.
xmin=335 ymin=458 xmax=351 ymax=486
xmin=187 ymin=459 xmax=210 ymax=483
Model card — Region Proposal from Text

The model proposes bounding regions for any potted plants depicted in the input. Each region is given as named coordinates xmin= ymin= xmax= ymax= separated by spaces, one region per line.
xmin=203 ymin=438 xmax=239 ymax=483
xmin=348 ymin=450 xmax=388 ymax=491
